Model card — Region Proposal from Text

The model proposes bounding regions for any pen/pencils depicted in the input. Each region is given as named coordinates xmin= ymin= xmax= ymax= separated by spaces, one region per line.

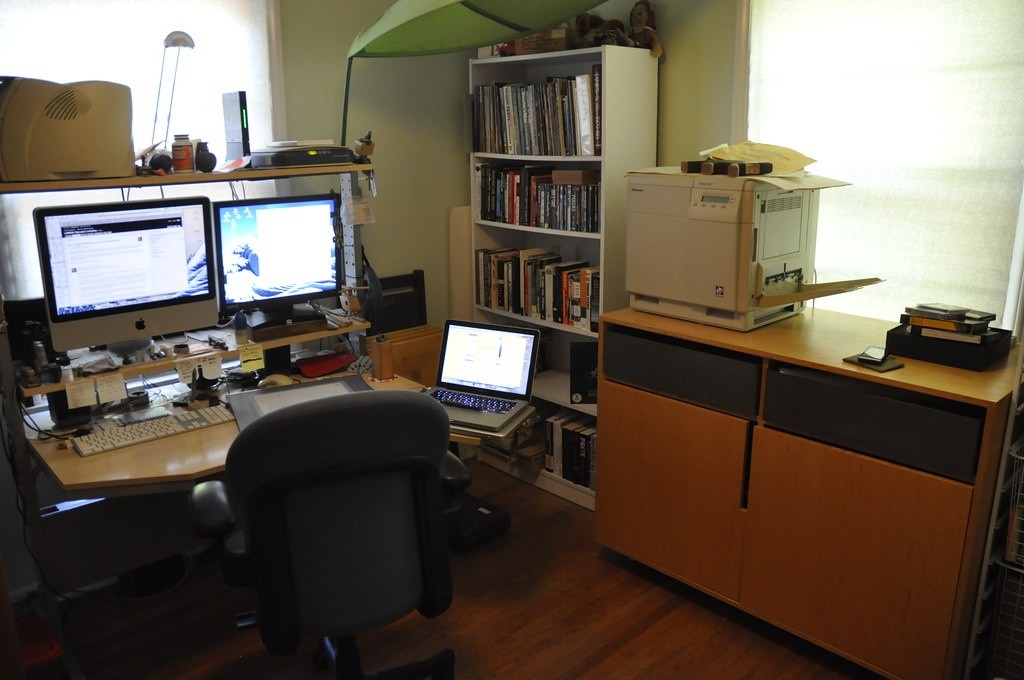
xmin=192 ymin=367 xmax=198 ymax=402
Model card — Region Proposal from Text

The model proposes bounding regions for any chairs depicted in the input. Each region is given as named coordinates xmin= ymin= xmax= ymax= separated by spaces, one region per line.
xmin=186 ymin=390 xmax=473 ymax=680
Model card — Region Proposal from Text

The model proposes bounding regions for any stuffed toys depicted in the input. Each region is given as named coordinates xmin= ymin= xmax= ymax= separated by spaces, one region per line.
xmin=577 ymin=0 xmax=662 ymax=59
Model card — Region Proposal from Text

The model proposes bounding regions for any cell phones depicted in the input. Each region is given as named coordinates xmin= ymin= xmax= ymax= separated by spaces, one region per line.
xmin=858 ymin=345 xmax=890 ymax=365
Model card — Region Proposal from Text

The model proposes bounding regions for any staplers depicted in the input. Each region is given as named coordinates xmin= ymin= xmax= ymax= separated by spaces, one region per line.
xmin=18 ymin=367 xmax=42 ymax=388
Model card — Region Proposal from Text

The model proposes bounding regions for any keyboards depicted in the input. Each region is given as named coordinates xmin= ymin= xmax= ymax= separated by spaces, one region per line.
xmin=71 ymin=405 xmax=236 ymax=457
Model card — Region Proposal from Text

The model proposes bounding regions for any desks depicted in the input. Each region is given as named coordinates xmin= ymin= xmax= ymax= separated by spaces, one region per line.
xmin=19 ymin=308 xmax=484 ymax=680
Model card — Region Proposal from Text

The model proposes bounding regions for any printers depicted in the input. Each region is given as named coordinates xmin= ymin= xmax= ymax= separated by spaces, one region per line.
xmin=627 ymin=173 xmax=821 ymax=333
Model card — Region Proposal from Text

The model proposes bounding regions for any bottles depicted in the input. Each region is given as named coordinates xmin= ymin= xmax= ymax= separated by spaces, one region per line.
xmin=32 ymin=340 xmax=47 ymax=366
xmin=172 ymin=134 xmax=195 ymax=174
xmin=235 ymin=312 xmax=249 ymax=344
xmin=57 ymin=356 xmax=74 ymax=383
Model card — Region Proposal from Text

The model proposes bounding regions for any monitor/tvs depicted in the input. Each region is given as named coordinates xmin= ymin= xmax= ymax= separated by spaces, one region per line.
xmin=33 ymin=196 xmax=219 ymax=362
xmin=214 ymin=192 xmax=342 ymax=330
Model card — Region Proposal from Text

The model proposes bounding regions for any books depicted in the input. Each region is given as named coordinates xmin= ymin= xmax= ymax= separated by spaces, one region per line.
xmin=569 ymin=340 xmax=598 ymax=404
xmin=474 ymin=246 xmax=600 ymax=333
xmin=472 ymin=63 xmax=602 ymax=156
xmin=544 ymin=412 xmax=597 ymax=491
xmin=475 ymin=162 xmax=600 ymax=234
xmin=899 ymin=302 xmax=1001 ymax=343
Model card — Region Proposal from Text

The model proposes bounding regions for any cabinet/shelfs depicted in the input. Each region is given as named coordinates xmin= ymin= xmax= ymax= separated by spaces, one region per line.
xmin=595 ymin=304 xmax=1024 ymax=680
xmin=468 ymin=44 xmax=658 ymax=511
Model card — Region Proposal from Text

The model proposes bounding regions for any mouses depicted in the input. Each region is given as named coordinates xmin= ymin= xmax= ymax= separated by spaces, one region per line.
xmin=258 ymin=374 xmax=293 ymax=388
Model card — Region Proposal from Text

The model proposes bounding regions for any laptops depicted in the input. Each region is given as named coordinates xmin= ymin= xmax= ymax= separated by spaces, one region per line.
xmin=421 ymin=319 xmax=542 ymax=431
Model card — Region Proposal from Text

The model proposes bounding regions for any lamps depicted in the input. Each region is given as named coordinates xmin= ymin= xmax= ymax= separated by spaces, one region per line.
xmin=144 ymin=31 xmax=196 ymax=172
